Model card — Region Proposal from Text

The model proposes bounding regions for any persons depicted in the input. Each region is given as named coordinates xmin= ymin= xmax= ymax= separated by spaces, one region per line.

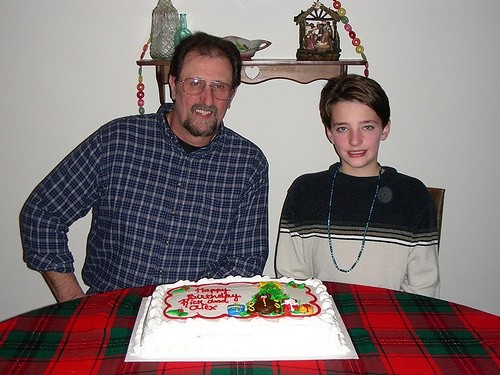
xmin=274 ymin=73 xmax=440 ymax=299
xmin=18 ymin=31 xmax=269 ymax=304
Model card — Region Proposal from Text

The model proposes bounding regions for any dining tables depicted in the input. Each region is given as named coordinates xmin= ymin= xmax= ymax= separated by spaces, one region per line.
xmin=0 ymin=280 xmax=500 ymax=375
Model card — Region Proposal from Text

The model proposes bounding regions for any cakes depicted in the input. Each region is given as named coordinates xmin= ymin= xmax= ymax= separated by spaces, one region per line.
xmin=129 ymin=274 xmax=351 ymax=361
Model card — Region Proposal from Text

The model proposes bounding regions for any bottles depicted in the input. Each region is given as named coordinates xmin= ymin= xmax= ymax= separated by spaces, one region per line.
xmin=150 ymin=0 xmax=179 ymax=61
xmin=174 ymin=14 xmax=192 ymax=49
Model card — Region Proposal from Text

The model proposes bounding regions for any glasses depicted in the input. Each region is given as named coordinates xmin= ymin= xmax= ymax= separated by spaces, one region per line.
xmin=175 ymin=73 xmax=235 ymax=101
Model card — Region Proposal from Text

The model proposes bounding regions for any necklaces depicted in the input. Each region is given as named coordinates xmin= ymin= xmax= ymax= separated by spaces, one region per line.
xmin=327 ymin=162 xmax=381 ymax=273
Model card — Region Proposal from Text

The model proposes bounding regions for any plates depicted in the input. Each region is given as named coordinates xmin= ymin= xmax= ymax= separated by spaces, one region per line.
xmin=221 ymin=36 xmax=273 ymax=61
